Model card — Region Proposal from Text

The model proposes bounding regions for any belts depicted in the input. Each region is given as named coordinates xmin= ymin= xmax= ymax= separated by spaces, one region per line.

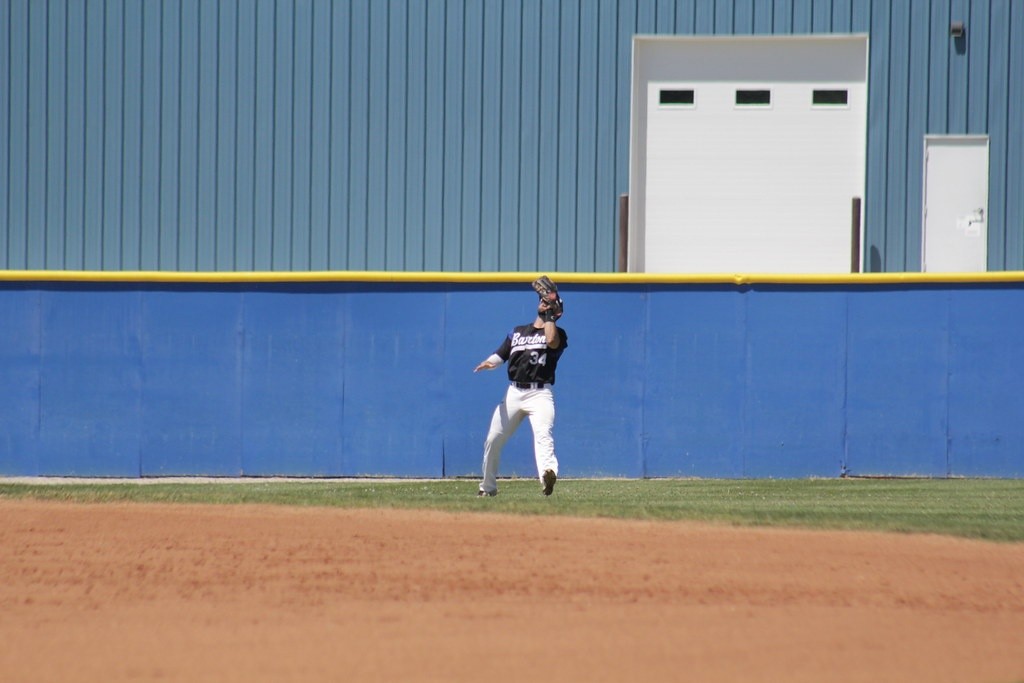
xmin=511 ymin=382 xmax=544 ymax=389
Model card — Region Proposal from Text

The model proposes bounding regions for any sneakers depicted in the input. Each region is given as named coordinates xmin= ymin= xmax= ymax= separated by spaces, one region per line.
xmin=479 ymin=489 xmax=497 ymax=497
xmin=542 ymin=469 xmax=557 ymax=497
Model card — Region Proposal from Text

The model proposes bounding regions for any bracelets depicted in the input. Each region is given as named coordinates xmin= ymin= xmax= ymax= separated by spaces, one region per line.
xmin=545 ymin=309 xmax=556 ymax=323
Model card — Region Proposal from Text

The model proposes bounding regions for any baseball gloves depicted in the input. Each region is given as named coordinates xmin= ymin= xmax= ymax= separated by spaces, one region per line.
xmin=530 ymin=275 xmax=564 ymax=323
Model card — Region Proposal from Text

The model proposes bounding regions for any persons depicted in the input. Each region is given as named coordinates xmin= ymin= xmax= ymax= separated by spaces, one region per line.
xmin=474 ymin=276 xmax=568 ymax=498
